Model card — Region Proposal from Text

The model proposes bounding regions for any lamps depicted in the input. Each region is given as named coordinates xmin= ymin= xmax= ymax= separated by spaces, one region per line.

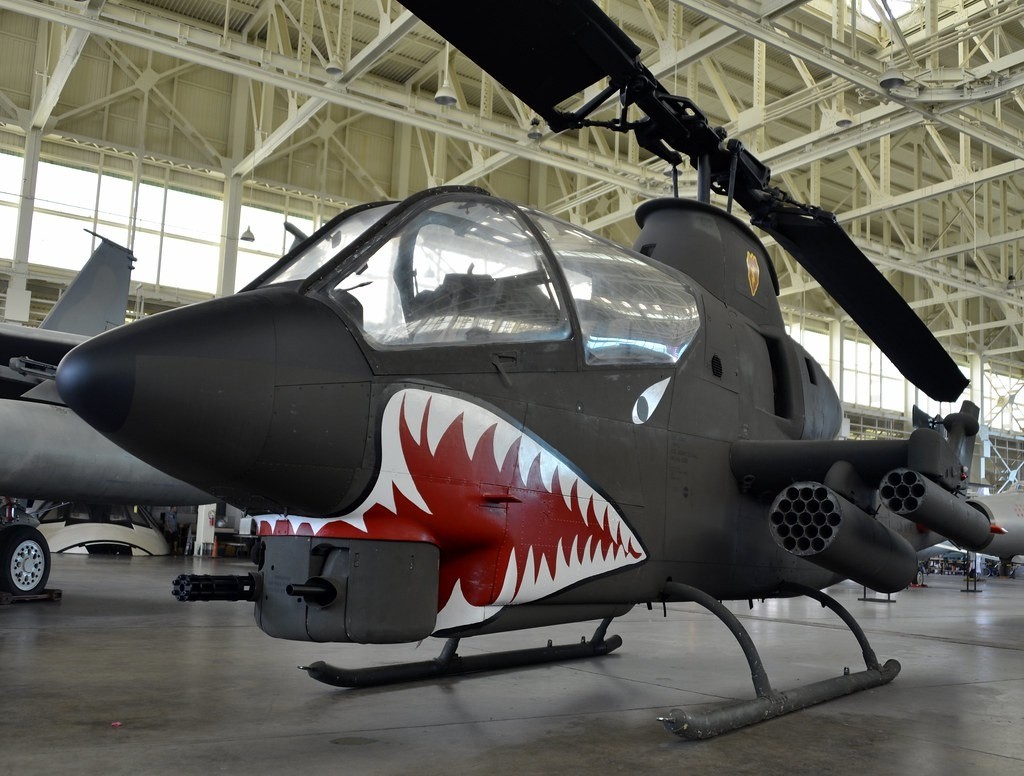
xmin=325 ymin=55 xmax=343 ymax=76
xmin=240 ymin=165 xmax=255 ymax=242
xmin=527 ymin=108 xmax=543 ymax=139
xmin=880 ymin=0 xmax=905 ymax=89
xmin=835 ymin=105 xmax=853 ymax=128
xmin=434 ymin=41 xmax=458 ymax=107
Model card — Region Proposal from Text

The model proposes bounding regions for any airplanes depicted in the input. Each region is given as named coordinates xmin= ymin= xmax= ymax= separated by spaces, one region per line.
xmin=0 ymin=228 xmax=223 ymax=608
xmin=56 ymin=0 xmax=1024 ymax=740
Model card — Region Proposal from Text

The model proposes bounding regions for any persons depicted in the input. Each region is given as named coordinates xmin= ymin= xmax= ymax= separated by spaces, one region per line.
xmin=164 ymin=506 xmax=179 ymax=553
